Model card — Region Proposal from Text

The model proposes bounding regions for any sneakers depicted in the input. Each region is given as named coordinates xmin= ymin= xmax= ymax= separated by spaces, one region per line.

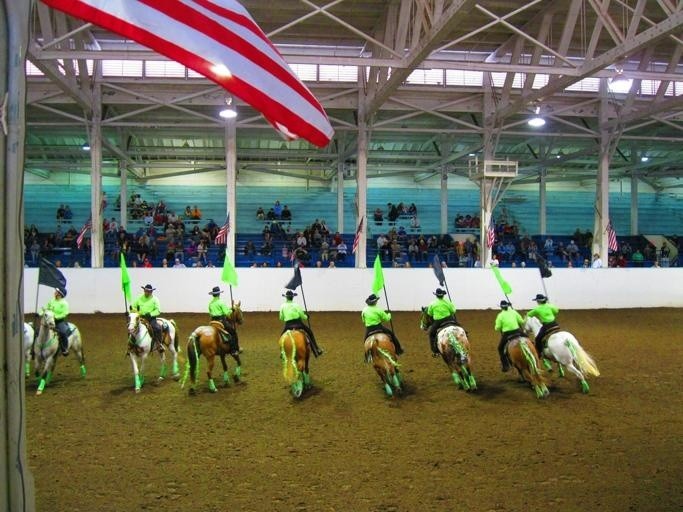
xmin=315 ymin=349 xmax=322 ymax=356
xmin=231 ymin=350 xmax=239 ymax=356
xmin=397 ymin=350 xmax=402 ymax=354
xmin=62 ymin=348 xmax=68 ymax=355
xmin=431 ymin=352 xmax=440 ymax=357
xmin=502 ymin=366 xmax=509 ymax=372
xmin=157 ymin=343 xmax=164 ymax=352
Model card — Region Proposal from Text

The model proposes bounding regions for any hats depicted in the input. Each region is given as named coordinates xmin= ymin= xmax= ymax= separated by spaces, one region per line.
xmin=365 ymin=294 xmax=380 ymax=304
xmin=500 ymin=301 xmax=511 ymax=307
xmin=282 ymin=290 xmax=297 ymax=296
xmin=141 ymin=284 xmax=155 ymax=291
xmin=209 ymin=287 xmax=223 ymax=295
xmin=433 ymin=288 xmax=446 ymax=295
xmin=55 ymin=286 xmax=66 ymax=297
xmin=532 ymin=294 xmax=547 ymax=302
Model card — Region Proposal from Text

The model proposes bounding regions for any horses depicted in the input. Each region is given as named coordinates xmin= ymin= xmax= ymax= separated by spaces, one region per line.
xmin=277 ymin=329 xmax=311 ymax=397
xmin=126 ymin=305 xmax=182 ymax=393
xmin=362 ymin=332 xmax=405 ymax=397
xmin=505 ymin=327 xmax=550 ymax=400
xmin=420 ymin=306 xmax=477 ymax=393
xmin=24 ymin=322 xmax=39 ymax=379
xmin=522 ymin=311 xmax=602 ymax=394
xmin=178 ymin=300 xmax=244 ymax=397
xmin=33 ymin=307 xmax=87 ymax=396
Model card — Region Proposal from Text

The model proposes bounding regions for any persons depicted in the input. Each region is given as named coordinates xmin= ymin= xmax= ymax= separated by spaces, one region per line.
xmin=427 ymin=289 xmax=460 ymax=358
xmin=374 ymin=203 xmax=421 ymax=232
xmin=244 ymin=205 xmax=347 ymax=268
xmin=608 ymin=234 xmax=683 ymax=268
xmin=528 ymin=294 xmax=560 ymax=358
xmin=34 ymin=286 xmax=70 ymax=357
xmin=361 ymin=294 xmax=404 ymax=355
xmin=125 ymin=285 xmax=164 ymax=354
xmin=497 ymin=208 xmax=513 ymax=228
xmin=24 ymin=190 xmax=219 ymax=267
xmin=494 ymin=301 xmax=525 ymax=372
xmin=455 ymin=212 xmax=481 ymax=232
xmin=279 ymin=290 xmax=323 ymax=358
xmin=592 ymin=253 xmax=603 ymax=267
xmin=208 ymin=286 xmax=243 ymax=356
xmin=377 ymin=221 xmax=593 ymax=268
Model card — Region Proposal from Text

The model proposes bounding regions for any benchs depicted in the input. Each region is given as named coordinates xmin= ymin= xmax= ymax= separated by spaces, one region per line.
xmin=21 ymin=183 xmax=683 ymax=268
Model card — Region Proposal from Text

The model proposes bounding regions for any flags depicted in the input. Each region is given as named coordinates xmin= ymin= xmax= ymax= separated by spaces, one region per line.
xmin=372 ymin=254 xmax=385 ymax=296
xmin=39 ymin=257 xmax=66 ymax=288
xmin=352 ymin=218 xmax=363 ymax=254
xmin=121 ymin=253 xmax=131 ymax=301
xmin=606 ymin=219 xmax=617 ymax=252
xmin=221 ymin=249 xmax=238 ymax=287
xmin=490 ymin=264 xmax=513 ymax=296
xmin=215 ymin=212 xmax=230 ymax=244
xmin=38 ymin=0 xmax=336 ymax=150
xmin=535 ymin=252 xmax=552 ymax=278
xmin=432 ymin=254 xmax=445 ymax=287
xmin=286 ymin=258 xmax=302 ymax=290
